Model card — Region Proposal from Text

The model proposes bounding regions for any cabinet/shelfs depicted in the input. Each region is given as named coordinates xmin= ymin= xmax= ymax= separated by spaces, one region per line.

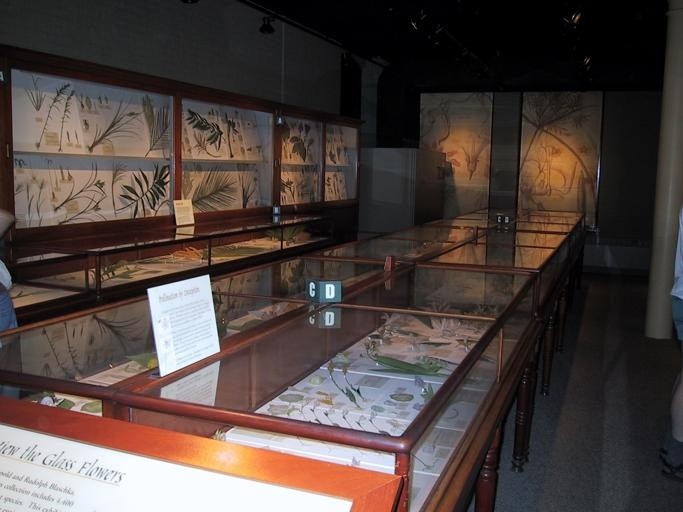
xmin=0 ymin=255 xmax=413 ymax=419
xmin=114 ymin=263 xmax=539 ymax=512
xmin=411 ymin=229 xmax=569 ymax=395
xmin=6 ymin=215 xmax=332 ymax=325
xmin=298 ymin=224 xmax=493 ymax=262
